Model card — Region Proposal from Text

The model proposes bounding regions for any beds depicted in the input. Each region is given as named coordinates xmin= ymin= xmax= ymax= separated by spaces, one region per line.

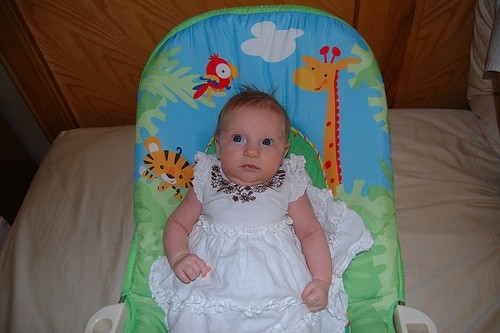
xmin=2 ymin=107 xmax=500 ymax=333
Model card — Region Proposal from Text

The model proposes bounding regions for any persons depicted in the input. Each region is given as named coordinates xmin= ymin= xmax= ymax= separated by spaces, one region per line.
xmin=148 ymin=83 xmax=375 ymax=333
